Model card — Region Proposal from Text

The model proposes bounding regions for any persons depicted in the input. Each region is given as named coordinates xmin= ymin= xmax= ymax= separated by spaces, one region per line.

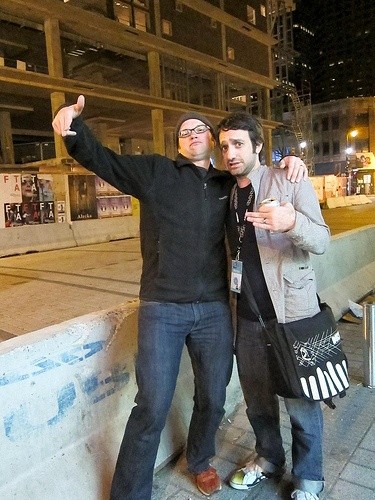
xmin=21 ymin=178 xmax=34 ymax=203
xmin=52 ymin=95 xmax=308 ymax=500
xmin=216 ymin=109 xmax=331 ymax=500
xmin=77 ymin=180 xmax=90 ymax=213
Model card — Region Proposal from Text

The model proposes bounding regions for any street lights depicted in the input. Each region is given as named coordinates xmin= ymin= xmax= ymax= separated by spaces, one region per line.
xmin=345 ymin=130 xmax=358 ymax=177
xmin=35 ymin=143 xmax=49 ymax=160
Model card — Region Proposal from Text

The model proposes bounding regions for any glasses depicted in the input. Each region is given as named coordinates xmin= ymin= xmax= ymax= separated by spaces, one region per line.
xmin=177 ymin=124 xmax=210 ymax=138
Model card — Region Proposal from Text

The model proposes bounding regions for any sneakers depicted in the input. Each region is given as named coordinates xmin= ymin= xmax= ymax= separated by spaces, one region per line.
xmin=292 ymin=488 xmax=319 ymax=500
xmin=196 ymin=464 xmax=222 ymax=496
xmin=229 ymin=461 xmax=268 ymax=489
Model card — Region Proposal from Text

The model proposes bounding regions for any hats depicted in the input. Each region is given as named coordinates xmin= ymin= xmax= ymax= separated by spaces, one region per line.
xmin=174 ymin=112 xmax=218 ymax=148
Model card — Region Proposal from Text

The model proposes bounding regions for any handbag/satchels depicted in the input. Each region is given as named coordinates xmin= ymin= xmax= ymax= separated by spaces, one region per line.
xmin=262 ymin=302 xmax=351 ymax=402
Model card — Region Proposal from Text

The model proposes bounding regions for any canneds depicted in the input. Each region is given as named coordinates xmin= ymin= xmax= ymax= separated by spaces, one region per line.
xmin=261 ymin=197 xmax=282 ymax=235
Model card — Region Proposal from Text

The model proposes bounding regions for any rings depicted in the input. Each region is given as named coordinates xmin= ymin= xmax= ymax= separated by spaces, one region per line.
xmin=264 ymin=218 xmax=267 ymax=223
